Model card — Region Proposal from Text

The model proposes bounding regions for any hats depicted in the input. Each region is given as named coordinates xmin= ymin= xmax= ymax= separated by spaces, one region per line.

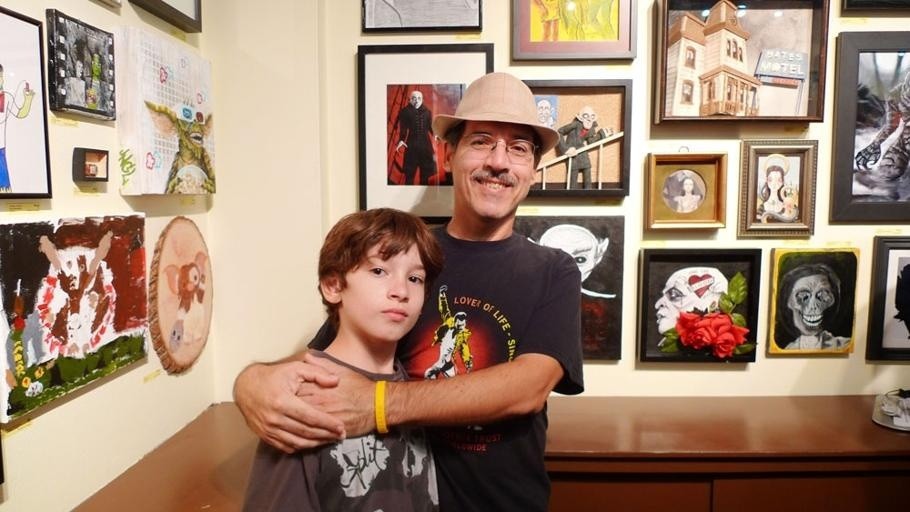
xmin=432 ymin=72 xmax=560 ymax=156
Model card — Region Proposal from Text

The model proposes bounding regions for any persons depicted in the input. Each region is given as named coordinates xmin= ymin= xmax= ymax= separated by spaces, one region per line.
xmin=395 ymin=90 xmax=442 ymax=186
xmin=559 ymin=105 xmax=613 ymax=190
xmin=243 ymin=208 xmax=440 ymax=511
xmin=232 ymin=74 xmax=582 ymax=512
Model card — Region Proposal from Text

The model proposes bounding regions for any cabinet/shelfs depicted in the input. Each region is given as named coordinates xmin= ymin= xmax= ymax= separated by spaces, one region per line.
xmin=544 ymin=396 xmax=910 ymax=512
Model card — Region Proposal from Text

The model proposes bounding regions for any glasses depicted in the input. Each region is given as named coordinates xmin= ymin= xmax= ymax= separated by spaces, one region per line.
xmin=462 ymin=134 xmax=540 ymax=159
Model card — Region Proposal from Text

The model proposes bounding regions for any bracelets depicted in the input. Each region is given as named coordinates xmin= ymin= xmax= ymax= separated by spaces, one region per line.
xmin=376 ymin=382 xmax=391 ymax=434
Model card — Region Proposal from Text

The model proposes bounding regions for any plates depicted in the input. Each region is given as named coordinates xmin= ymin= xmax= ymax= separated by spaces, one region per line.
xmin=872 ymin=394 xmax=910 ymax=431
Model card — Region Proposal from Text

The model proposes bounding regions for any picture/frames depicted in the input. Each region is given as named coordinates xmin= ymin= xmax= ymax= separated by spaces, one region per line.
xmin=765 ymin=248 xmax=860 ymax=360
xmin=521 ymin=78 xmax=633 ymax=196
xmin=0 ymin=6 xmax=52 ymax=199
xmin=646 ymin=153 xmax=728 ymax=232
xmin=509 ymin=0 xmax=637 ymax=61
xmin=362 ymin=0 xmax=483 ymax=32
xmin=128 ymin=0 xmax=202 ymax=33
xmin=635 ymin=248 xmax=762 ymax=363
xmin=865 ymin=236 xmax=910 ymax=360
xmin=738 ymin=139 xmax=819 ymax=239
xmin=650 ymin=0 xmax=826 ymax=126
xmin=828 ymin=31 xmax=910 ymax=225
xmin=358 ymin=43 xmax=494 ymax=226
xmin=841 ymin=0 xmax=910 ymax=17
xmin=46 ymin=8 xmax=116 ymax=120
